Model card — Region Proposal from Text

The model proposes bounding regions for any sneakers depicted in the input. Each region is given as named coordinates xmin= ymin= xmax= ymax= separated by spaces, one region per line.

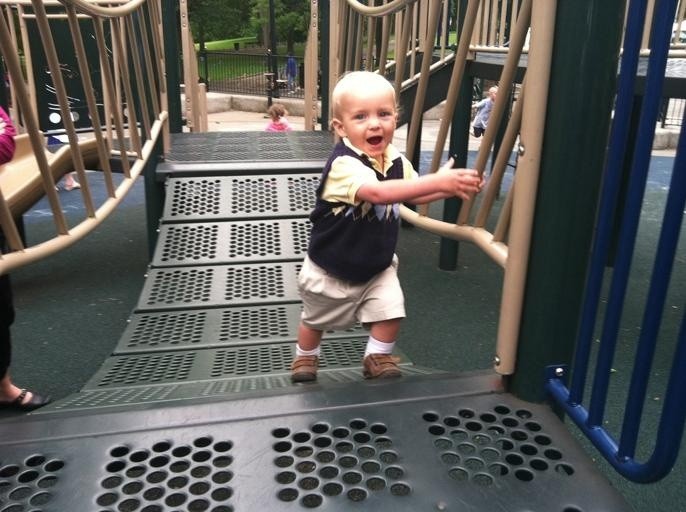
xmin=291 ymin=356 xmax=319 ymax=381
xmin=363 ymin=353 xmax=402 ymax=379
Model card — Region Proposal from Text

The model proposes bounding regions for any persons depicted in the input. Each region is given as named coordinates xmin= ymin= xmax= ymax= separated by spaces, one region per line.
xmin=0 ymin=104 xmax=51 ymax=409
xmin=290 ymin=71 xmax=485 ymax=381
xmin=265 ymin=103 xmax=292 ymax=131
xmin=286 ymin=51 xmax=296 ymax=94
xmin=469 ymin=86 xmax=499 ymax=137
xmin=64 ymin=170 xmax=81 ymax=191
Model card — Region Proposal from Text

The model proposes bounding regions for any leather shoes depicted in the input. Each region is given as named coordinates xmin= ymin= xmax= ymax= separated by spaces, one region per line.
xmin=1 ymin=389 xmax=53 ymax=410
